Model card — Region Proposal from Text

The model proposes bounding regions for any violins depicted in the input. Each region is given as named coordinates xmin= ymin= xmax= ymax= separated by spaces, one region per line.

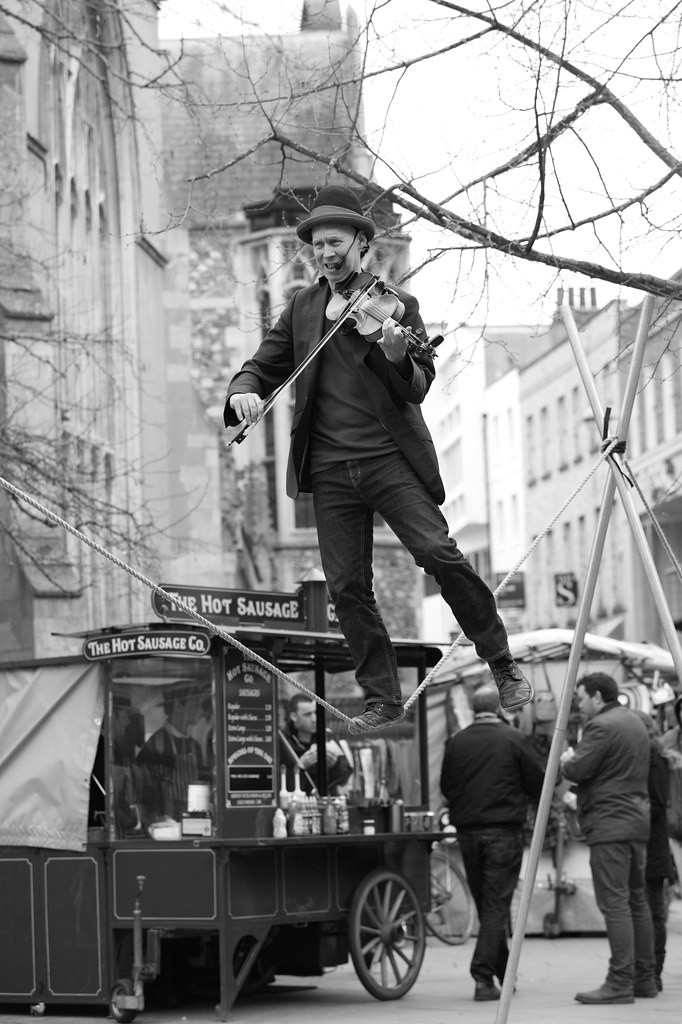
xmin=325 ymin=271 xmax=445 ymax=361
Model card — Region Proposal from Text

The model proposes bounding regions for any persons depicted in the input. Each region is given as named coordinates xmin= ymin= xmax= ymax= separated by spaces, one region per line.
xmin=224 ymin=184 xmax=532 ymax=735
xmin=278 ymin=693 xmax=351 ymax=797
xmin=86 ymin=680 xmax=209 ymax=829
xmin=439 ymin=683 xmax=546 ymax=1000
xmin=558 ymin=672 xmax=682 ymax=1003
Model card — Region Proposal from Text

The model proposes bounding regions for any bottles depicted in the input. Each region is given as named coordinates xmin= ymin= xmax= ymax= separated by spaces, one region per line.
xmin=273 ymin=764 xmax=349 ymax=838
xmin=362 ymin=819 xmax=377 ymax=834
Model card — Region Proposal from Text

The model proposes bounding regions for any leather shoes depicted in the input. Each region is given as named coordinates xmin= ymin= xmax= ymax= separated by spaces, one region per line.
xmin=348 ymin=703 xmax=406 ymax=736
xmin=488 ymin=650 xmax=534 ymax=713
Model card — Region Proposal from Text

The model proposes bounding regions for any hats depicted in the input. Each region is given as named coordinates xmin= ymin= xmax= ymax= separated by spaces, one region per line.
xmin=155 ymin=681 xmax=211 ymax=706
xmin=112 ymin=691 xmax=140 ymax=712
xmin=297 ymin=185 xmax=374 ymax=246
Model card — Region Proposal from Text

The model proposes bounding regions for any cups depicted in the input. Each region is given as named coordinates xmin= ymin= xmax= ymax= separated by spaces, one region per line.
xmin=389 ymin=803 xmax=402 ymax=833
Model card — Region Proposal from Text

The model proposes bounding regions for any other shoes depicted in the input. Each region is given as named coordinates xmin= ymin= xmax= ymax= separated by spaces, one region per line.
xmin=656 ymin=980 xmax=663 ymax=991
xmin=631 ymin=984 xmax=657 ymax=998
xmin=475 ymin=985 xmax=500 ymax=1000
xmin=574 ymin=987 xmax=635 ymax=1004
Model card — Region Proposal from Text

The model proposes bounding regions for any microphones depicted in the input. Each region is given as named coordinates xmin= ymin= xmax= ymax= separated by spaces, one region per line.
xmin=334 ymin=230 xmax=360 ymax=269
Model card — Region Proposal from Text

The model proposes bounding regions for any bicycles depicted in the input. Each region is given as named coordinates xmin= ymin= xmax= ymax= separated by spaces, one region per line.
xmin=420 ymin=846 xmax=476 ymax=946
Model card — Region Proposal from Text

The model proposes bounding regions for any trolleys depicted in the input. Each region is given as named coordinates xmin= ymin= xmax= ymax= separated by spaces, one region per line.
xmin=84 ymin=580 xmax=461 ymax=1022
